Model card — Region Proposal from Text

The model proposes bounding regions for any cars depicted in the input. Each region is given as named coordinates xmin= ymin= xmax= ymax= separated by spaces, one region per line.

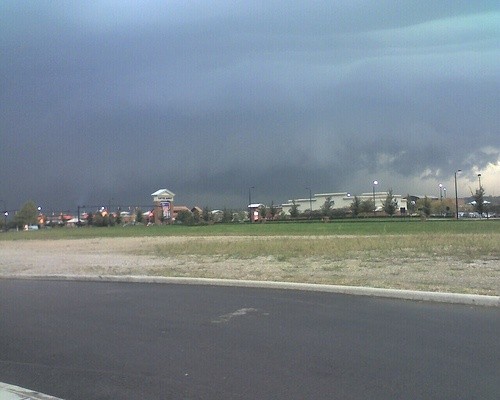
xmin=469 ymin=212 xmax=496 ymax=218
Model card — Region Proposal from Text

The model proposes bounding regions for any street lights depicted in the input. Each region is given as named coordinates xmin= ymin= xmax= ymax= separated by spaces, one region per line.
xmin=478 ymin=174 xmax=482 ymax=187
xmin=372 ymin=180 xmax=379 ymax=213
xmin=249 ymin=185 xmax=256 ymax=223
xmin=443 ymin=188 xmax=446 ymax=199
xmin=454 ymin=169 xmax=465 ymax=219
xmin=439 ymin=184 xmax=443 ymax=204
